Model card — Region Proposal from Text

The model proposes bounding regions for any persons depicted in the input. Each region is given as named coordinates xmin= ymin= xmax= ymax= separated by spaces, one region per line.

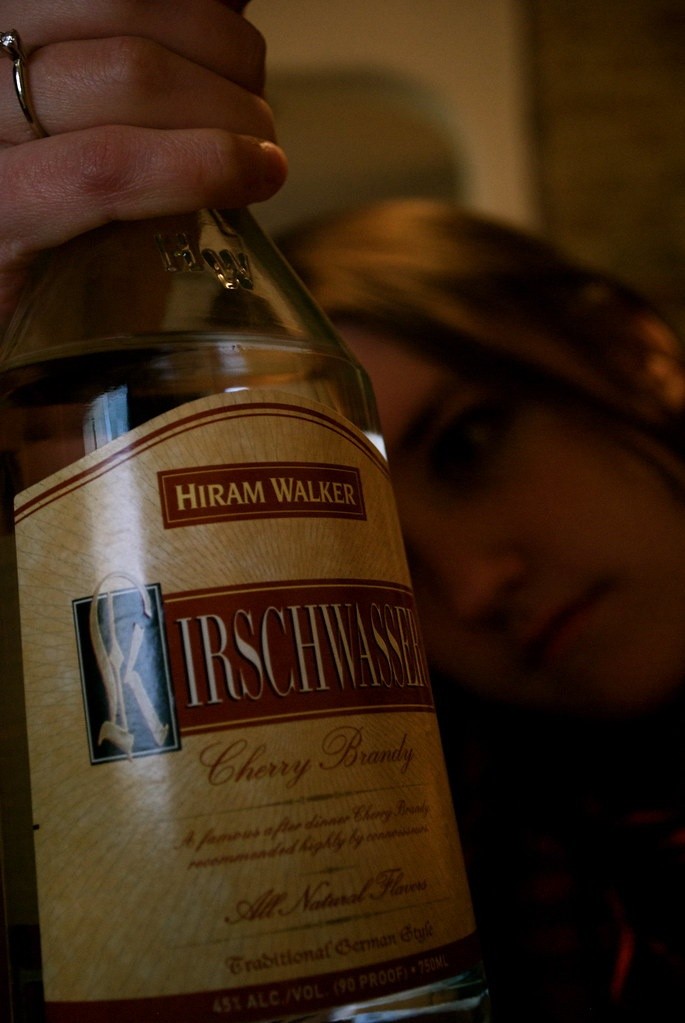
xmin=0 ymin=0 xmax=684 ymax=1023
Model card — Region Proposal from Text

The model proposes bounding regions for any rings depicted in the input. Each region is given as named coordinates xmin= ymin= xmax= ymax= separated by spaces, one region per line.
xmin=0 ymin=27 xmax=50 ymax=138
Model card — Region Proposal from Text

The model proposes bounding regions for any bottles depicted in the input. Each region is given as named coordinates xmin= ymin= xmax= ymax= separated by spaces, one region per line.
xmin=0 ymin=200 xmax=493 ymax=1023
